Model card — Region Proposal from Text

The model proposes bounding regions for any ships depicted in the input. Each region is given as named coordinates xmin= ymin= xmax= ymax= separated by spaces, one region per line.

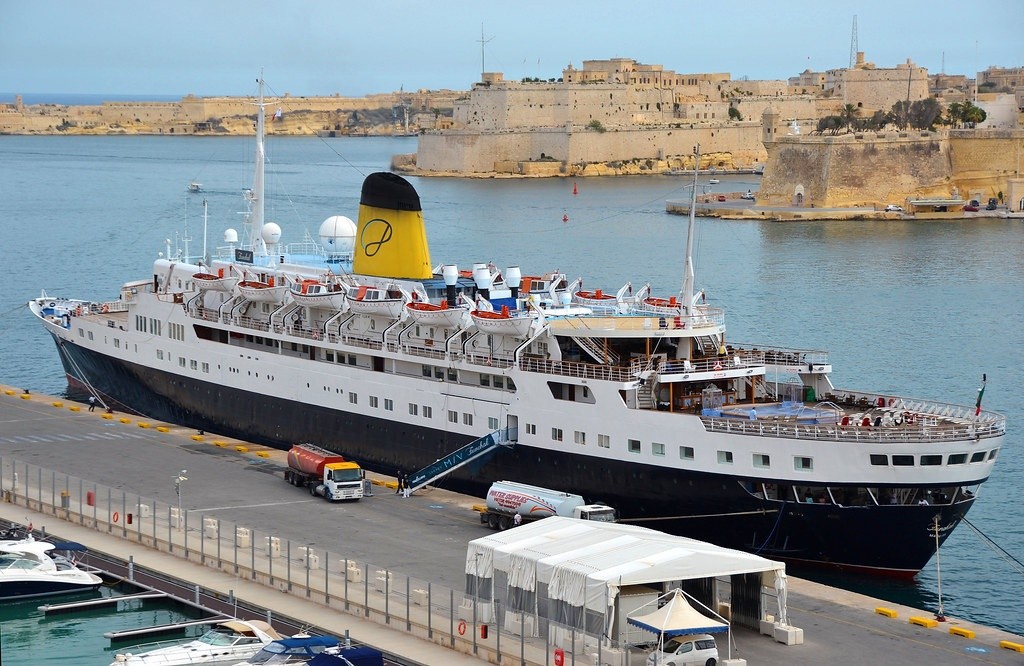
xmin=26 ymin=59 xmax=1010 ymax=585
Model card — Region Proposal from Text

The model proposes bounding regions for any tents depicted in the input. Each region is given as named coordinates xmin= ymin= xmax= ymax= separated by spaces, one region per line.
xmin=461 ymin=515 xmax=788 ymax=650
xmin=626 ymin=588 xmax=731 ymax=666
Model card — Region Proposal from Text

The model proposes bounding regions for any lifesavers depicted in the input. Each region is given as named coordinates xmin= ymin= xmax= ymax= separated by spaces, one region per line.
xmin=713 ymin=361 xmax=722 ymax=370
xmin=50 ymin=302 xmax=55 ymax=307
xmin=113 ymin=512 xmax=118 ymax=522
xmin=488 ymin=358 xmax=493 ymax=366
xmin=202 ymin=309 xmax=207 ymax=319
xmin=458 ymin=621 xmax=467 ymax=635
xmin=102 ymin=305 xmax=109 ymax=312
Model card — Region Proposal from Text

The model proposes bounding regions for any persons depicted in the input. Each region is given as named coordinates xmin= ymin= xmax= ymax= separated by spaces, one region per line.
xmin=401 ymin=474 xmax=409 ymax=498
xmin=513 ymin=511 xmax=522 ymax=526
xmin=716 ymin=341 xmax=728 ymax=361
xmin=395 ymin=471 xmax=404 ymax=493
xmin=749 ymin=407 xmax=758 ymax=427
xmin=88 ymin=394 xmax=97 ymax=412
xmin=64 ymin=307 xmax=71 ymax=320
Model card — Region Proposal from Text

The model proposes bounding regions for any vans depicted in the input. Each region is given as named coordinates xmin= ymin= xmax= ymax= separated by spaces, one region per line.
xmin=970 ymin=200 xmax=979 ymax=207
xmin=646 ymin=634 xmax=720 ymax=666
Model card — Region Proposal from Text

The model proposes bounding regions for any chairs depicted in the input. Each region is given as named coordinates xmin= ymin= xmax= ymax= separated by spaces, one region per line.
xmin=703 ymin=400 xmax=997 ymax=437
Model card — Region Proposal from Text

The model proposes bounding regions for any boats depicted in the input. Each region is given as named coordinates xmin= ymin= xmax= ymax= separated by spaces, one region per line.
xmin=190 ymin=267 xmax=239 ymax=294
xmin=342 ymin=282 xmax=409 ymax=320
xmin=237 ymin=273 xmax=290 ymax=306
xmin=575 ymin=288 xmax=618 ymax=309
xmin=643 ymin=295 xmax=687 ymax=316
xmin=470 ymin=304 xmax=534 ymax=341
xmin=404 ymin=297 xmax=468 ymax=330
xmin=709 ymin=176 xmax=720 ymax=184
xmin=289 ymin=273 xmax=346 ymax=311
xmin=107 ymin=616 xmax=386 ymax=666
xmin=0 ymin=521 xmax=104 ymax=605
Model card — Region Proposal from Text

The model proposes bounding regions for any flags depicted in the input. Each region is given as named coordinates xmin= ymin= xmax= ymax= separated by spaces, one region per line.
xmin=272 ymin=107 xmax=282 ymax=121
xmin=976 ymin=385 xmax=985 ymax=414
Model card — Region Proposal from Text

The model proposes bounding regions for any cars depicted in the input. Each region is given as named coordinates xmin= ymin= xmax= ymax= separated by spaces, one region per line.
xmin=718 ymin=195 xmax=725 ymax=202
xmin=884 ymin=204 xmax=902 ymax=212
xmin=963 ymin=204 xmax=980 ymax=212
xmin=986 ymin=203 xmax=996 ymax=210
xmin=741 ymin=192 xmax=755 ymax=200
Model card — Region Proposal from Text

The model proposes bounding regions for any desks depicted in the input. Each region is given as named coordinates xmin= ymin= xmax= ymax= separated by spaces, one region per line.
xmin=837 ymin=422 xmax=874 ymax=426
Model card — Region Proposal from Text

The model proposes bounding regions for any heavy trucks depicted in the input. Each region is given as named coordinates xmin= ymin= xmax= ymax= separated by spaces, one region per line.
xmin=472 ymin=480 xmax=623 ymax=531
xmin=284 ymin=442 xmax=366 ymax=503
